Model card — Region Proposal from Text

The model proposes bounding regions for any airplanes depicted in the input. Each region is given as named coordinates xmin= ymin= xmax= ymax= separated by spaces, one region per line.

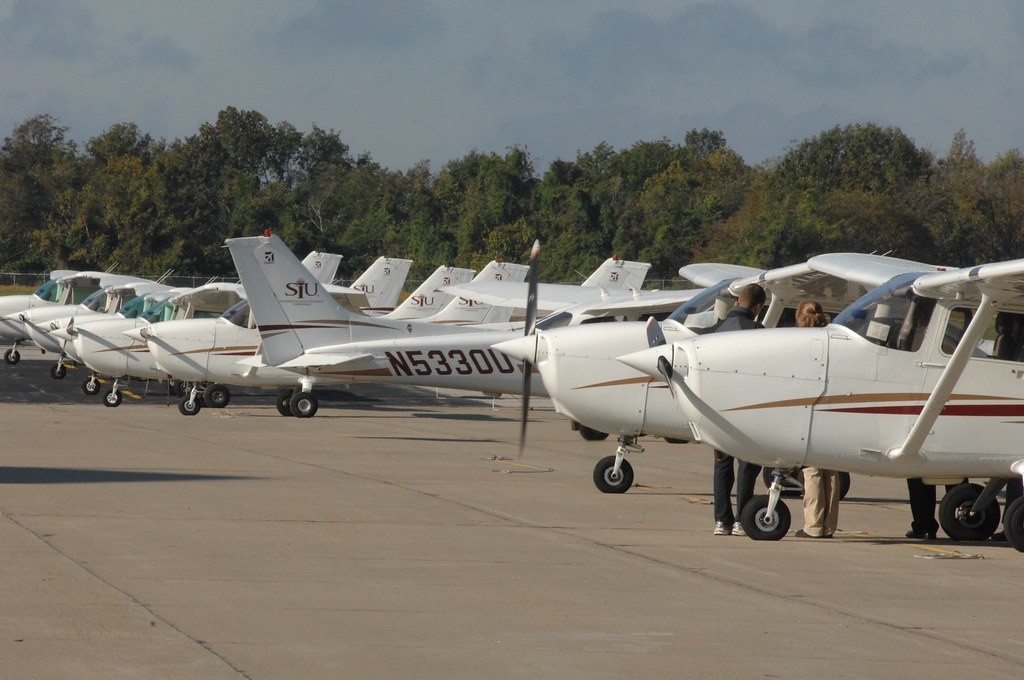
xmin=0 ymin=229 xmax=653 ymax=417
xmin=494 ymin=251 xmax=996 ymax=502
xmin=613 ymin=257 xmax=1024 ymax=551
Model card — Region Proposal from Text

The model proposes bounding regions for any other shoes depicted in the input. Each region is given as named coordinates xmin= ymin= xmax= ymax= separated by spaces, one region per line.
xmin=823 ymin=535 xmax=834 ymax=539
xmin=906 ymin=520 xmax=939 ymax=539
xmin=795 ymin=529 xmax=822 ymax=538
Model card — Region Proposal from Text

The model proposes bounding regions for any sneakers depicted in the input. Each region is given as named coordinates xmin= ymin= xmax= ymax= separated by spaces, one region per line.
xmin=732 ymin=522 xmax=746 ymax=536
xmin=713 ymin=521 xmax=728 ymax=536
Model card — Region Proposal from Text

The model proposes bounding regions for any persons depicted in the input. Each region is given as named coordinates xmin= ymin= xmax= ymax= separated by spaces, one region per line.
xmin=906 ymin=477 xmax=968 ymax=540
xmin=990 ymin=478 xmax=1023 ymax=542
xmin=713 ymin=283 xmax=767 ymax=537
xmin=793 ymin=300 xmax=841 ymax=539
